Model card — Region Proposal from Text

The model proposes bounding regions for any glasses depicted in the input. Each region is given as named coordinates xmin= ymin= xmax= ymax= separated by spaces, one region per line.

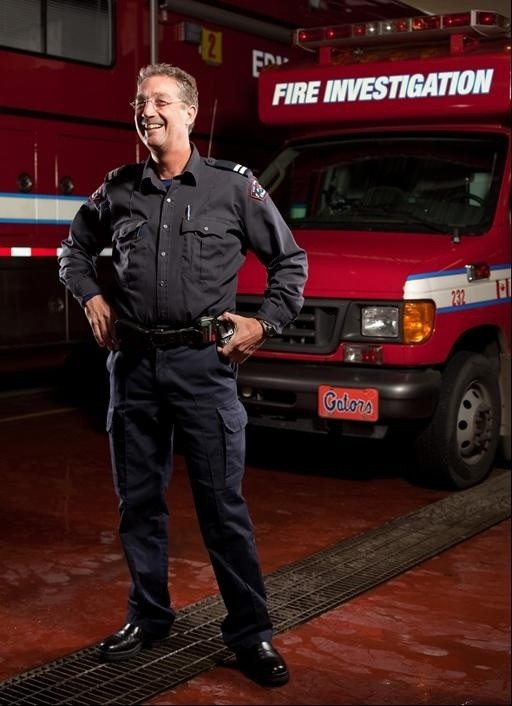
xmin=130 ymin=98 xmax=183 ymax=107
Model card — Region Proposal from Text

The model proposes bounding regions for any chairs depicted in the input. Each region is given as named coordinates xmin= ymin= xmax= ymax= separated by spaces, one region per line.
xmin=322 ymin=159 xmax=474 ymax=223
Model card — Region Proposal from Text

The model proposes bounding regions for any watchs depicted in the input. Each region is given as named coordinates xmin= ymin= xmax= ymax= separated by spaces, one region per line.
xmin=258 ymin=319 xmax=277 ymax=339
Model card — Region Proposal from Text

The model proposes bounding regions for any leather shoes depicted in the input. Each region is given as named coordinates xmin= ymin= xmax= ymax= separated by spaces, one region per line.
xmin=99 ymin=622 xmax=172 ymax=662
xmin=221 ymin=634 xmax=289 ymax=686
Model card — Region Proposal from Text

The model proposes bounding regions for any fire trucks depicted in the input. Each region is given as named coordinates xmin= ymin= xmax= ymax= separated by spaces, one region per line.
xmin=223 ymin=10 xmax=510 ymax=489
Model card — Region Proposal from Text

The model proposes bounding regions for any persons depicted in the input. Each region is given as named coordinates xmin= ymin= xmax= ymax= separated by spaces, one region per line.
xmin=58 ymin=63 xmax=310 ymax=689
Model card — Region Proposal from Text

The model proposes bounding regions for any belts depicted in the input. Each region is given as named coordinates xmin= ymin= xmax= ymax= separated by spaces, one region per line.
xmin=112 ymin=320 xmax=235 ymax=348
